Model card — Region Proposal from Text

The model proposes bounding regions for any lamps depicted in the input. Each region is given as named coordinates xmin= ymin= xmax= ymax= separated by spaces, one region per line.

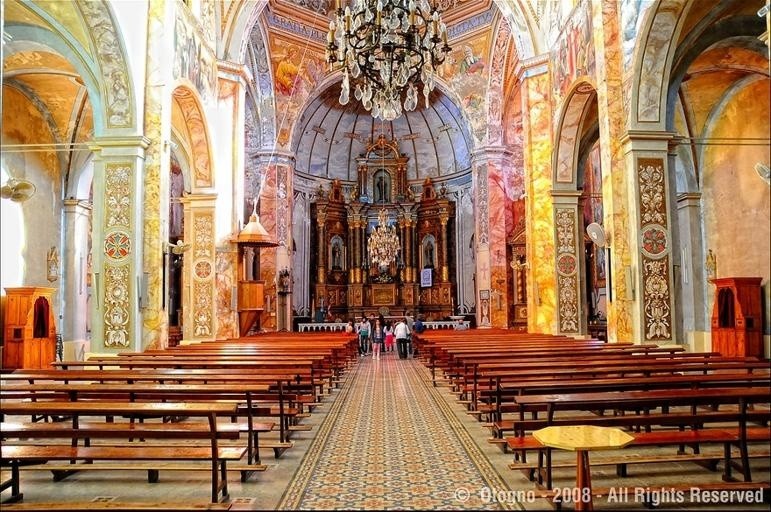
xmin=510 ymin=260 xmax=530 ymax=269
xmin=163 ymin=240 xmax=191 ymax=256
xmin=366 ymin=120 xmax=402 ymax=265
xmin=323 ymin=0 xmax=448 ymax=123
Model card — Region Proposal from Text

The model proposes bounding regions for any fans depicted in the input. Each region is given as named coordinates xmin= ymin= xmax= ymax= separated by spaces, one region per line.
xmin=1 ymin=179 xmax=37 ymax=202
xmin=755 ymin=163 xmax=770 ymax=181
xmin=228 ymin=213 xmax=279 ymax=281
xmin=586 ymin=223 xmax=610 ymax=248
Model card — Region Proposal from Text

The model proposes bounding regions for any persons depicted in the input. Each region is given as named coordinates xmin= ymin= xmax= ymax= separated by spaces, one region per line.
xmin=460 ymin=45 xmax=481 ymax=75
xmin=377 ymin=176 xmax=388 ymax=200
xmin=346 ymin=312 xmax=426 ymax=359
xmin=425 ymin=240 xmax=435 ymax=265
xmin=454 ymin=319 xmax=468 ymax=330
xmin=332 ymin=242 xmax=342 ymax=267
xmin=275 ymin=46 xmax=304 ymax=95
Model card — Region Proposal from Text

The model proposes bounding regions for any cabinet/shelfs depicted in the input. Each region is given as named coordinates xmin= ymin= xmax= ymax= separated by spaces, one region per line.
xmin=710 ymin=277 xmax=764 ymax=358
xmin=4 ymin=286 xmax=57 ymax=371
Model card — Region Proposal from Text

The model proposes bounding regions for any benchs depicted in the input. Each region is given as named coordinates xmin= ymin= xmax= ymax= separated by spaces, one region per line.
xmin=0 ymin=332 xmax=359 ymax=511
xmin=409 ymin=328 xmax=770 ymax=512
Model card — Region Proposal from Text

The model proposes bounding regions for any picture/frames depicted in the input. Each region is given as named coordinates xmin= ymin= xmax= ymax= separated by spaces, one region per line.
xmin=420 ymin=268 xmax=433 ymax=287
xmin=480 ymin=290 xmax=490 ymax=299
